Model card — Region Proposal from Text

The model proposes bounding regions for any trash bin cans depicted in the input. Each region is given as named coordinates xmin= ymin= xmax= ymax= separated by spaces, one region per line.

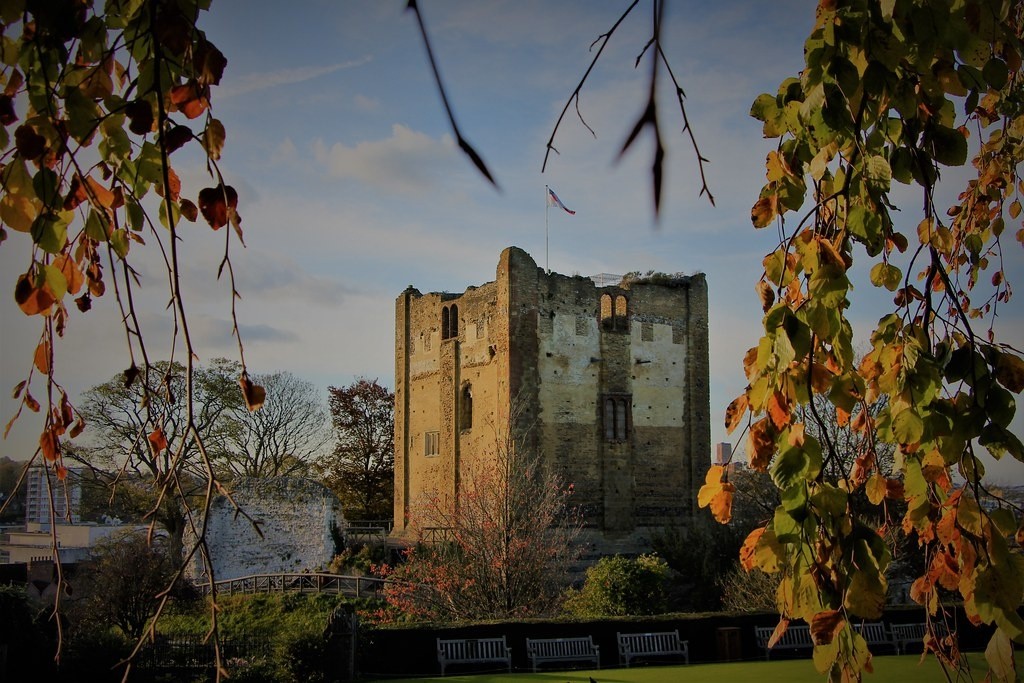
xmin=718 ymin=626 xmax=743 ymax=661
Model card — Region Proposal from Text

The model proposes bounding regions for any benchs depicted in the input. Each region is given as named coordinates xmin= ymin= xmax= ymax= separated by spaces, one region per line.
xmin=616 ymin=630 xmax=690 ymax=669
xmin=753 ymin=625 xmax=815 ymax=660
xmin=852 ymin=621 xmax=900 ymax=656
xmin=890 ymin=619 xmax=959 ymax=654
xmin=436 ymin=635 xmax=514 ymax=676
xmin=526 ymin=635 xmax=600 ymax=673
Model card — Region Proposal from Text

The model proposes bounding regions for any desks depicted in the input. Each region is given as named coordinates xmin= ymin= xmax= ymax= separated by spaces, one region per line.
xmin=718 ymin=626 xmax=742 ymax=662
